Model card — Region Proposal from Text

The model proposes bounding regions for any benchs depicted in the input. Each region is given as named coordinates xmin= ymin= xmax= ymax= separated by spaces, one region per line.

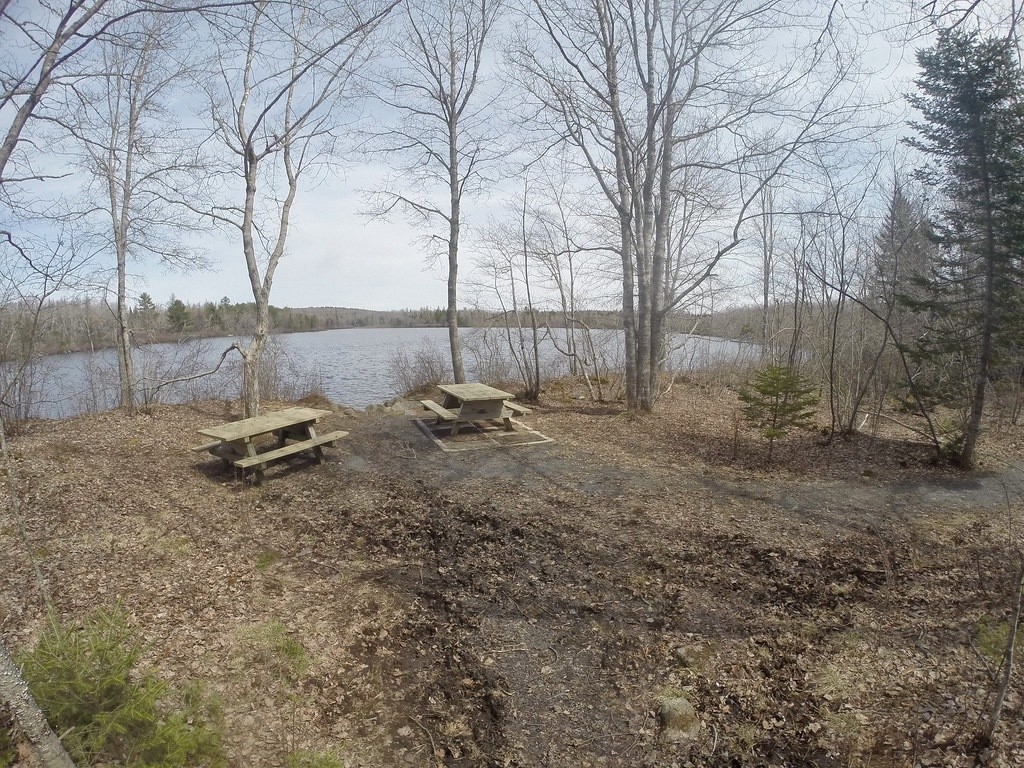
xmin=420 ymin=399 xmax=459 ymax=422
xmin=502 ymin=399 xmax=532 ymax=417
xmin=191 ymin=439 xmax=221 ymax=453
xmin=233 ymin=430 xmax=350 ymax=469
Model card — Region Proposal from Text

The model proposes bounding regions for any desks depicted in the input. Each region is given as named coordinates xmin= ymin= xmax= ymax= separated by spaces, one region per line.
xmin=198 ymin=405 xmax=334 ymax=489
xmin=435 ymin=382 xmax=516 ymax=437
